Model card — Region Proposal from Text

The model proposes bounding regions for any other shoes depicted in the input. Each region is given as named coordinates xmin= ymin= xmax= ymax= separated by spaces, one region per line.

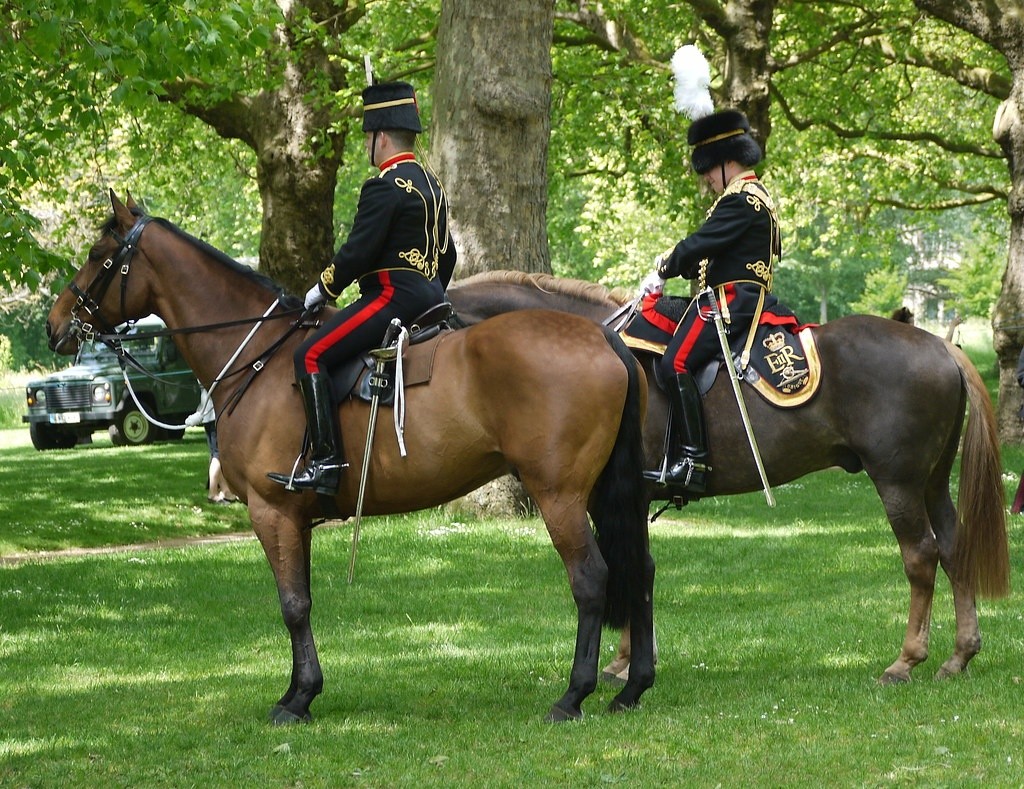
xmin=207 ymin=497 xmax=237 ymax=506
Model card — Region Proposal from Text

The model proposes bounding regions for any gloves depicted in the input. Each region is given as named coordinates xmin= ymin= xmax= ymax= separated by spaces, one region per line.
xmin=640 ymin=271 xmax=666 ymax=295
xmin=304 ymin=283 xmax=323 ymax=312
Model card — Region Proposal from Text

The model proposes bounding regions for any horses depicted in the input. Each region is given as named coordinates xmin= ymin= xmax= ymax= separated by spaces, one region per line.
xmin=39 ymin=187 xmax=1014 ymax=732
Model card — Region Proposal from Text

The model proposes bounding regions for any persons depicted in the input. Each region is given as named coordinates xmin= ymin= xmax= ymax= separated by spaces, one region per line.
xmin=891 ymin=307 xmax=915 ymax=328
xmin=199 ymin=387 xmax=242 ymax=504
xmin=639 ymin=110 xmax=781 ymax=494
xmin=266 ymin=82 xmax=457 ymax=496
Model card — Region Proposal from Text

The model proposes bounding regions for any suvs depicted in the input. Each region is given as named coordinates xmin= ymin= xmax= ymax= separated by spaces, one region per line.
xmin=21 ymin=319 xmax=201 ymax=450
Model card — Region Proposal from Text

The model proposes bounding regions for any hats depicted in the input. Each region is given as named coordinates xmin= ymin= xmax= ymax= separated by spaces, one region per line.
xmin=688 ymin=111 xmax=762 ymax=175
xmin=361 ymin=82 xmax=422 ymax=133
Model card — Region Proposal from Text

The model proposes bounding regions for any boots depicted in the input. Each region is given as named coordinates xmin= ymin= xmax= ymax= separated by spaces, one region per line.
xmin=266 ymin=372 xmax=344 ymax=496
xmin=643 ymin=373 xmax=708 ymax=492
